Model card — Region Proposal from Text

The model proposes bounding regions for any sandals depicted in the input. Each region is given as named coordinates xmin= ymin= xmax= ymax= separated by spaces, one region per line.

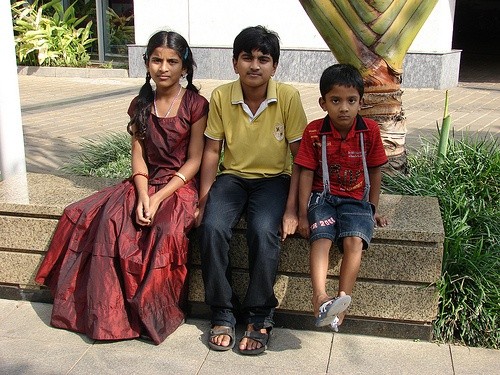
xmin=239 ymin=314 xmax=276 ymax=355
xmin=209 ymin=324 xmax=237 ymax=350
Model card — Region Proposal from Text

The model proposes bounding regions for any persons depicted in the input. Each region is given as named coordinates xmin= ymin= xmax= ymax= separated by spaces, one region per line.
xmin=35 ymin=31 xmax=210 ymax=347
xmin=294 ymin=63 xmax=388 ymax=333
xmin=196 ymin=25 xmax=309 ymax=355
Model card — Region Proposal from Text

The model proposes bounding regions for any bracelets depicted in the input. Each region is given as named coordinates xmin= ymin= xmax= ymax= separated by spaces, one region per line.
xmin=175 ymin=172 xmax=188 ymax=185
xmin=132 ymin=172 xmax=149 ymax=180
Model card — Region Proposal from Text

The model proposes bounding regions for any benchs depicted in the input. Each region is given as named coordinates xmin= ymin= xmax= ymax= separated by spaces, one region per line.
xmin=0 ymin=171 xmax=443 ymax=338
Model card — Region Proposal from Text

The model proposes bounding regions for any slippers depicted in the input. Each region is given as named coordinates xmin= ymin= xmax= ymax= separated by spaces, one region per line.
xmin=327 ymin=316 xmax=339 ymax=332
xmin=315 ymin=295 xmax=352 ymax=330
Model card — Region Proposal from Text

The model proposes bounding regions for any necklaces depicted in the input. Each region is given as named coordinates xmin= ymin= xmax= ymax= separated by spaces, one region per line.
xmin=153 ymin=83 xmax=182 ymax=118
